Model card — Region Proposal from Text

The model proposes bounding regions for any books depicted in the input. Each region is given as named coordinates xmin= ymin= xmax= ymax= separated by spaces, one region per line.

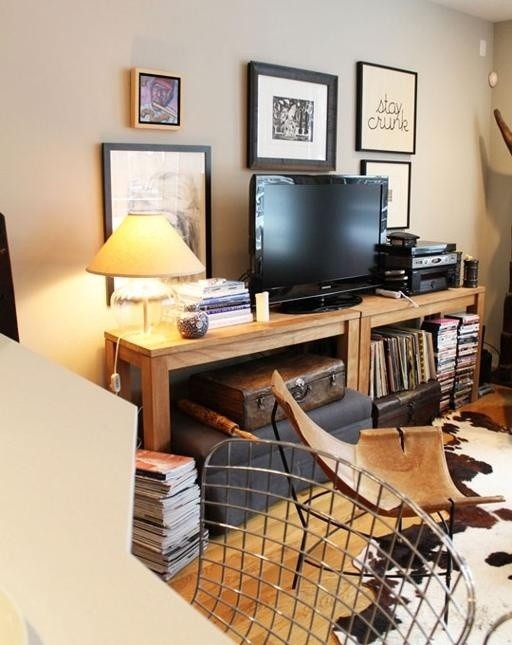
xmin=132 ymin=449 xmax=210 ymax=582
xmin=170 ymin=279 xmax=253 ymax=329
xmin=370 ymin=311 xmax=480 ymax=413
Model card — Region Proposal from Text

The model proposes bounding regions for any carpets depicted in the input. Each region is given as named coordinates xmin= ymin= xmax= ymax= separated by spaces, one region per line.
xmin=329 ymin=397 xmax=512 ymax=645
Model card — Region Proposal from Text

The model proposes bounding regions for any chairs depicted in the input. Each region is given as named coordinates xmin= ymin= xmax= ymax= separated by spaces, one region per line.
xmin=254 ymin=362 xmax=507 ymax=591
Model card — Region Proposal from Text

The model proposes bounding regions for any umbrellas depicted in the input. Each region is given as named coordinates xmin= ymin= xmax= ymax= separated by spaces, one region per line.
xmin=178 ymin=399 xmax=260 ymax=445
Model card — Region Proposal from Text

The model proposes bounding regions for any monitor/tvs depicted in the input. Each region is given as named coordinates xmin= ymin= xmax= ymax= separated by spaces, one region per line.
xmin=248 ymin=173 xmax=389 ymax=314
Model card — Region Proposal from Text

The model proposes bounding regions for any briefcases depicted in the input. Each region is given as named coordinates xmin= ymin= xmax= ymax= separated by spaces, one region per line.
xmin=189 ymin=353 xmax=344 ymax=431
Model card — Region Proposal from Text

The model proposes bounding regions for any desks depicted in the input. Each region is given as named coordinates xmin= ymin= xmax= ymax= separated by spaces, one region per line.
xmin=104 ymin=308 xmax=360 ymax=453
xmin=347 ymin=285 xmax=485 ymax=403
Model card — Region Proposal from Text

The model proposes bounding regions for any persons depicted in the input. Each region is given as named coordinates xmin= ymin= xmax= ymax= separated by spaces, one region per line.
xmin=141 ymin=76 xmax=178 ymax=123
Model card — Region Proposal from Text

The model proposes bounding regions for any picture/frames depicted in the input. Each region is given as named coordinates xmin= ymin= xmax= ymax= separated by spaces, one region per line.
xmin=246 ymin=60 xmax=338 ymax=173
xmin=353 ymin=60 xmax=419 ymax=230
xmin=127 ymin=67 xmax=185 ymax=132
xmin=99 ymin=141 xmax=214 ymax=308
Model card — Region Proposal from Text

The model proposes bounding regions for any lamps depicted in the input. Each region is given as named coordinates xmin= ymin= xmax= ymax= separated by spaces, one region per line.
xmin=86 ymin=206 xmax=205 ymax=345
